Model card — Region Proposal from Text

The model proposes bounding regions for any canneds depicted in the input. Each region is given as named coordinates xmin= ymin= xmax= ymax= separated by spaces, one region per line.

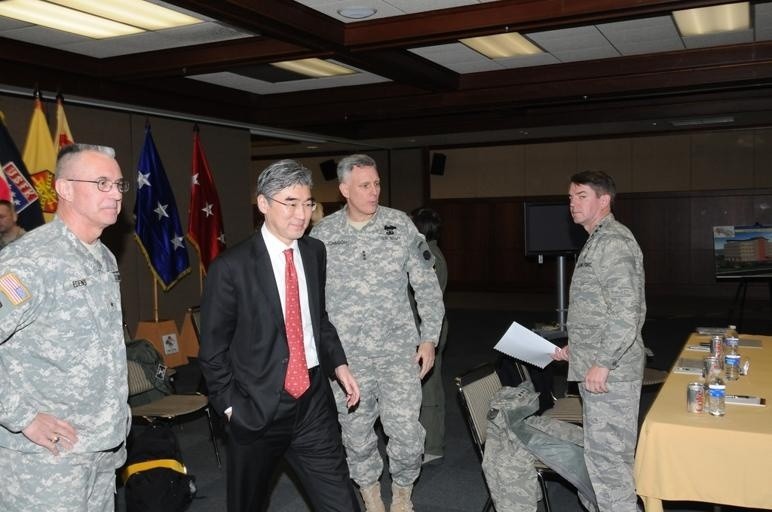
xmin=712 ymin=336 xmax=723 ymax=357
xmin=704 ymin=357 xmax=721 ymax=377
xmin=686 ymin=383 xmax=704 ymax=413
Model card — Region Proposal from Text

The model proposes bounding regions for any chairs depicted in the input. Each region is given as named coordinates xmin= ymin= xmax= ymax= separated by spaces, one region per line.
xmin=123 ymin=340 xmax=224 ymax=475
xmin=190 ymin=305 xmax=200 ymax=344
xmin=640 ymin=367 xmax=669 ymax=389
xmin=453 ymin=362 xmax=565 ymax=512
xmin=512 ymin=357 xmax=584 ymax=428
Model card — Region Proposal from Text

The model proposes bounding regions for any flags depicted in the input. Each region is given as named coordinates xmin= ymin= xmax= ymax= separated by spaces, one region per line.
xmin=188 ymin=127 xmax=228 ymax=278
xmin=131 ymin=116 xmax=190 ymax=291
xmin=23 ymin=88 xmax=59 ymax=223
xmin=52 ymin=91 xmax=76 ymax=153
xmin=0 ymin=110 xmax=43 ymax=234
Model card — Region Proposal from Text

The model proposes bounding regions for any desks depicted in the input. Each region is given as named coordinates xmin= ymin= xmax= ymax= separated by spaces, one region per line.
xmin=633 ymin=330 xmax=772 ymax=511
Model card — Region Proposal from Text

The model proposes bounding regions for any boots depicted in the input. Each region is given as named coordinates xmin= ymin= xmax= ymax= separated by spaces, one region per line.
xmin=390 ymin=484 xmax=415 ymax=512
xmin=359 ymin=481 xmax=386 ymax=512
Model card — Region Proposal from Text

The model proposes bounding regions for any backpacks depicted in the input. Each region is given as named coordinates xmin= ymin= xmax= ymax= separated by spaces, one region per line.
xmin=121 ymin=419 xmax=196 ymax=512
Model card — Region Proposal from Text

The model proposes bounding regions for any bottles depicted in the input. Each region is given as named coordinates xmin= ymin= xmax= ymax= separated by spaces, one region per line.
xmin=708 ymin=366 xmax=726 ymax=416
xmin=725 ymin=325 xmax=739 ymax=357
xmin=722 ymin=340 xmax=740 ymax=380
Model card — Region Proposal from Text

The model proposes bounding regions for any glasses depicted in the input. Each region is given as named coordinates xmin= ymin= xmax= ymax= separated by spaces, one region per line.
xmin=65 ymin=178 xmax=127 ymax=192
xmin=266 ymin=196 xmax=317 ymax=211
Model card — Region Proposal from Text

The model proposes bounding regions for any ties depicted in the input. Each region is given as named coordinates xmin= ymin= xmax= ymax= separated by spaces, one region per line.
xmin=283 ymin=249 xmax=310 ymax=399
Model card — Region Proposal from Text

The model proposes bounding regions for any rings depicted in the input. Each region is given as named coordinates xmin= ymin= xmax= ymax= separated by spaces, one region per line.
xmin=49 ymin=436 xmax=60 ymax=443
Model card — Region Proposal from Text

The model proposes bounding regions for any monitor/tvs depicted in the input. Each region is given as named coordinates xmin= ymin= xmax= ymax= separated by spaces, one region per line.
xmin=522 ymin=201 xmax=589 ymax=257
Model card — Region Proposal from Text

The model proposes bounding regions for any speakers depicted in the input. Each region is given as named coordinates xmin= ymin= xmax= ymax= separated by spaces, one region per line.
xmin=319 ymin=158 xmax=338 ymax=181
xmin=430 ymin=153 xmax=446 ymax=176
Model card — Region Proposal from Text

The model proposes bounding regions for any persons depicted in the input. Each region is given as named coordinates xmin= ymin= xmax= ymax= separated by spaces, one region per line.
xmin=308 ymin=154 xmax=448 ymax=511
xmin=0 ymin=198 xmax=28 ymax=247
xmin=553 ymin=170 xmax=649 ymax=512
xmin=0 ymin=143 xmax=130 ymax=512
xmin=407 ymin=208 xmax=447 ymax=463
xmin=197 ymin=160 xmax=362 ymax=512
